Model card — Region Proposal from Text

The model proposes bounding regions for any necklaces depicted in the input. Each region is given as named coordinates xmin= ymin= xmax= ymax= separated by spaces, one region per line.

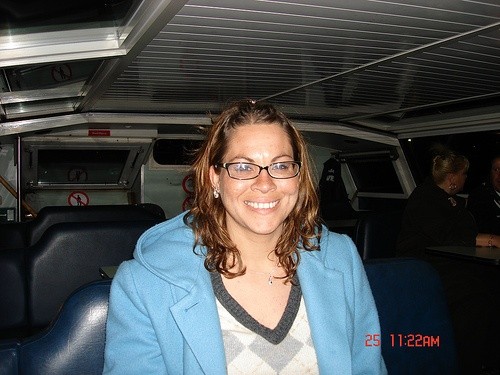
xmin=243 ymin=240 xmax=282 ymax=285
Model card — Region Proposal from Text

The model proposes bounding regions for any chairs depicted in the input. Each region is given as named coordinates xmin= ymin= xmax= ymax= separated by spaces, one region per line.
xmin=0 ymin=200 xmax=459 ymax=375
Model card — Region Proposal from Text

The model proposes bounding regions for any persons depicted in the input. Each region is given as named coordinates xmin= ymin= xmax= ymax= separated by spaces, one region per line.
xmin=396 ymin=151 xmax=500 ymax=255
xmin=104 ymin=102 xmax=390 ymax=374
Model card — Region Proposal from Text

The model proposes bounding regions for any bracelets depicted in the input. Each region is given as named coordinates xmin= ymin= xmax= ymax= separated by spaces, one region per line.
xmin=487 ymin=235 xmax=495 ymax=245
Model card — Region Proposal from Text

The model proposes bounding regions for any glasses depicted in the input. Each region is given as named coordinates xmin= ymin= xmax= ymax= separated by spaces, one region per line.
xmin=491 ymin=167 xmax=500 ymax=173
xmin=215 ymin=161 xmax=302 ymax=180
